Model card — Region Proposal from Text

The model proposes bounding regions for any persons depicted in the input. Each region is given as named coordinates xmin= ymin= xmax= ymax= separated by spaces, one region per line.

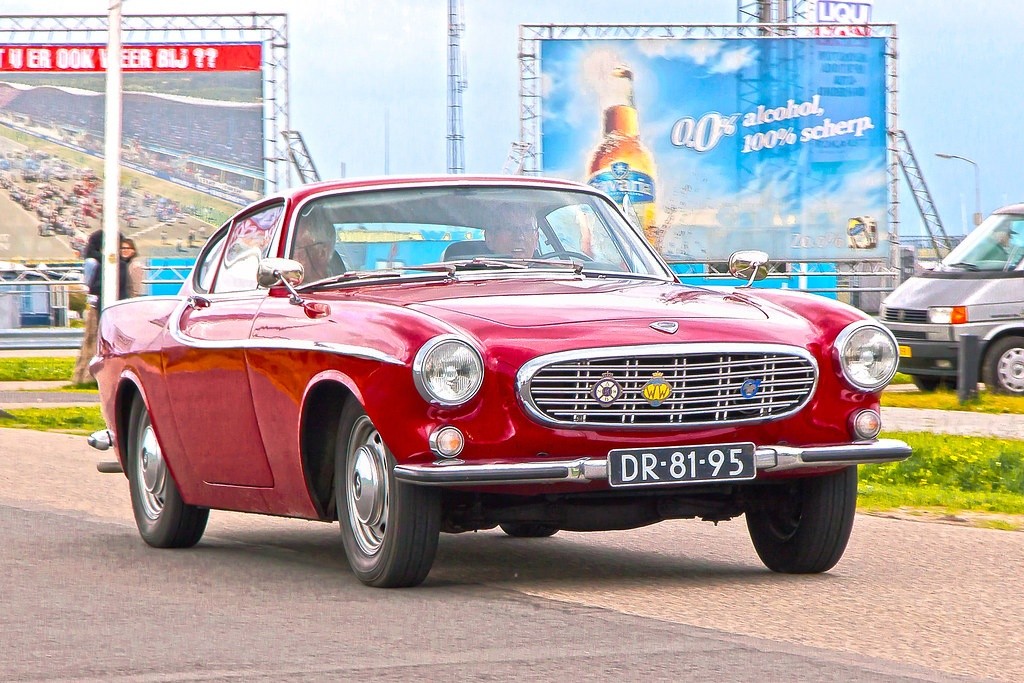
xmin=126 ymin=209 xmax=206 ymax=252
xmin=978 ymin=221 xmax=1011 ymax=259
xmin=38 ymin=209 xmax=58 ymax=236
xmin=294 ymin=210 xmax=336 ymax=284
xmin=485 ymin=202 xmax=540 ymax=266
xmin=74 ymin=218 xmax=142 ymax=383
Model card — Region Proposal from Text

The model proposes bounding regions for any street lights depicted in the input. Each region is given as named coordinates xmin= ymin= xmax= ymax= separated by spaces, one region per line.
xmin=936 ymin=152 xmax=982 ymax=228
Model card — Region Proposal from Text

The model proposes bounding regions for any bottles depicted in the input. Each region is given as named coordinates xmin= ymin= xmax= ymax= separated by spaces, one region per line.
xmin=586 ymin=105 xmax=659 ymax=254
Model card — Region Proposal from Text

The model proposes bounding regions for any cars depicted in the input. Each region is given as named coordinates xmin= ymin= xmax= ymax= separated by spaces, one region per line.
xmin=86 ymin=173 xmax=914 ymax=590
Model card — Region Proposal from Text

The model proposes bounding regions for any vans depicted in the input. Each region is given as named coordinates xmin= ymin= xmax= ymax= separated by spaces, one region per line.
xmin=878 ymin=200 xmax=1024 ymax=397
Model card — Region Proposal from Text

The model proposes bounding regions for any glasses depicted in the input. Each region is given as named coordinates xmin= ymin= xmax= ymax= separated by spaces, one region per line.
xmin=120 ymin=246 xmax=133 ymax=250
xmin=494 ymin=223 xmax=538 ymax=237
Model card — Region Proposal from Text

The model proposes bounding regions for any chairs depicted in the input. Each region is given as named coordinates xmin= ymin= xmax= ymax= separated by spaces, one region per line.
xmin=442 ymin=240 xmax=493 ymax=260
